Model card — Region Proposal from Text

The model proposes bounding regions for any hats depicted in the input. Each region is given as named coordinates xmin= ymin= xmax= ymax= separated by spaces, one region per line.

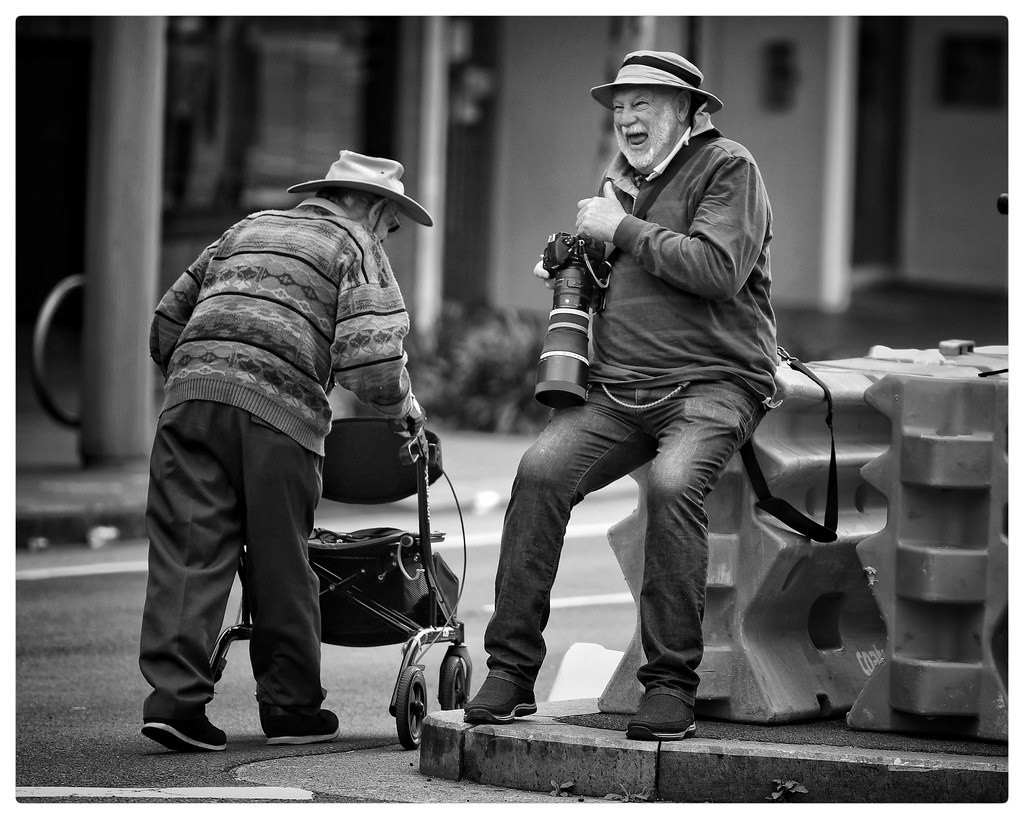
xmin=286 ymin=150 xmax=434 ymax=227
xmin=590 ymin=50 xmax=723 ymax=114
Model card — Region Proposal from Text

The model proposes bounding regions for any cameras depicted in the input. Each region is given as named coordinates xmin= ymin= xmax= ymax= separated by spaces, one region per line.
xmin=535 ymin=232 xmax=606 ymax=407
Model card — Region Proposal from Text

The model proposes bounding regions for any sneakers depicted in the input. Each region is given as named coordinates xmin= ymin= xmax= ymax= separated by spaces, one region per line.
xmin=141 ymin=714 xmax=227 ymax=751
xmin=464 ymin=677 xmax=537 ymax=725
xmin=627 ymin=695 xmax=696 ymax=741
xmin=261 ymin=709 xmax=340 ymax=745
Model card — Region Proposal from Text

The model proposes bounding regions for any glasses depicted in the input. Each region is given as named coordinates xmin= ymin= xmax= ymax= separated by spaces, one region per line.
xmin=386 ymin=202 xmax=401 ymax=233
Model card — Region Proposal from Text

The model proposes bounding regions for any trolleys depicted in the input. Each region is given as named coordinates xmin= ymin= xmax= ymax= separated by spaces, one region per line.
xmin=210 ymin=411 xmax=472 ymax=753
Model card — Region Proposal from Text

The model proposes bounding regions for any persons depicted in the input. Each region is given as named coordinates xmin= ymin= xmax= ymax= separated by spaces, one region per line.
xmin=464 ymin=50 xmax=779 ymax=742
xmin=141 ymin=149 xmax=434 ymax=751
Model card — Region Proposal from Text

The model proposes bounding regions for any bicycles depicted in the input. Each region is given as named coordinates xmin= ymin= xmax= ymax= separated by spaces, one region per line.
xmin=32 ymin=274 xmax=88 ymax=431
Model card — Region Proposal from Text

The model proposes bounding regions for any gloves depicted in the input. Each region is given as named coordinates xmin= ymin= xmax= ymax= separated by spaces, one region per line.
xmin=393 ymin=397 xmax=426 ymax=440
